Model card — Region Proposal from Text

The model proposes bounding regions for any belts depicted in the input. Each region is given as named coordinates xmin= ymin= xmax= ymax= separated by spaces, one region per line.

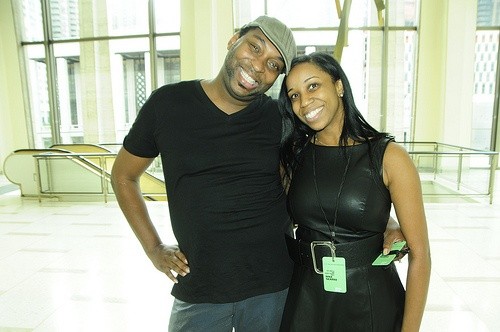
xmin=286 ymin=231 xmax=384 ymax=275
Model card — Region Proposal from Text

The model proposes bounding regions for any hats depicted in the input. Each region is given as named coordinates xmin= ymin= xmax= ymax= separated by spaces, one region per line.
xmin=242 ymin=16 xmax=297 ymax=76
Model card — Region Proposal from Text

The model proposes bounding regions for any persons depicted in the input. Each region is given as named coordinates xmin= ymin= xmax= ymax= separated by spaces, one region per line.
xmin=276 ymin=52 xmax=433 ymax=332
xmin=109 ymin=14 xmax=409 ymax=332
